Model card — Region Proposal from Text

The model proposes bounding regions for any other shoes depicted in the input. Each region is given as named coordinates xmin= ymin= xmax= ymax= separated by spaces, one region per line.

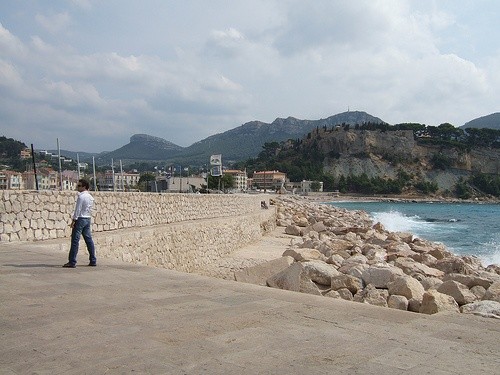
xmin=89 ymin=261 xmax=96 ymax=266
xmin=63 ymin=262 xmax=76 ymax=268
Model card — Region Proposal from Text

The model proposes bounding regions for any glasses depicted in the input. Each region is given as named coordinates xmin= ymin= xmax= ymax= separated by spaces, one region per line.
xmin=77 ymin=185 xmax=84 ymax=187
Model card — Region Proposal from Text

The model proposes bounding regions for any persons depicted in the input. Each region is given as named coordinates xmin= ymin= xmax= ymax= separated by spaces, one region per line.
xmin=207 ymin=190 xmax=210 ymax=194
xmin=62 ymin=179 xmax=99 ymax=267
xmin=125 ymin=185 xmax=129 ymax=192
xmin=196 ymin=189 xmax=200 ymax=194
